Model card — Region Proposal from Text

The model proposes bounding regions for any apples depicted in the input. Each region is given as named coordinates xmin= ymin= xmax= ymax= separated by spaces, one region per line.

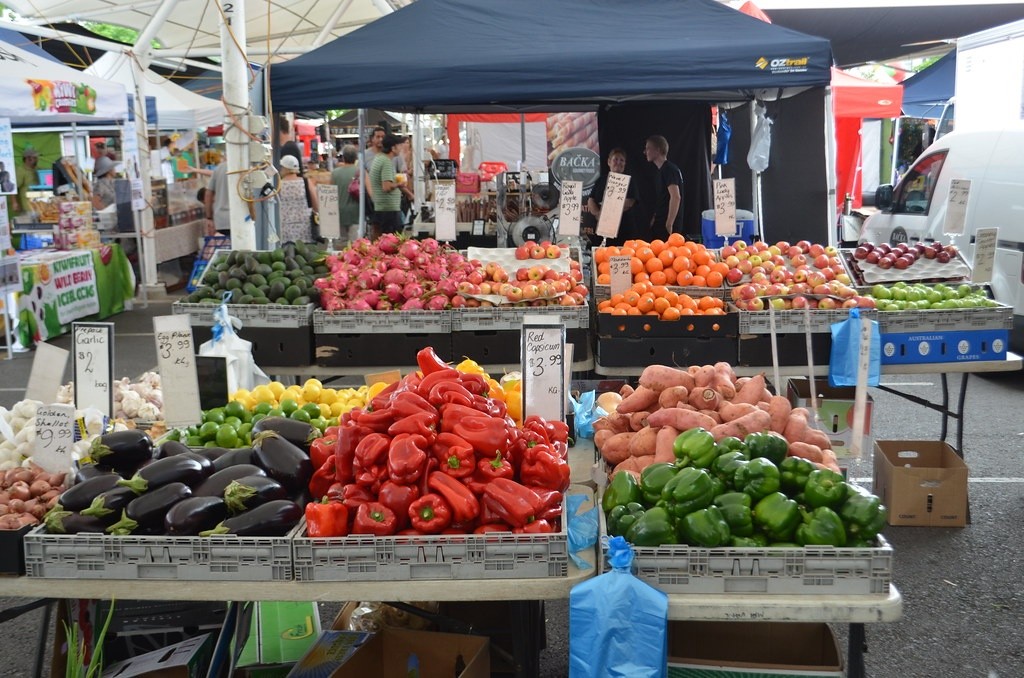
xmin=451 ymin=242 xmax=588 ymax=309
xmin=720 ymin=240 xmax=876 ymax=311
xmin=854 ymin=241 xmax=997 ymax=309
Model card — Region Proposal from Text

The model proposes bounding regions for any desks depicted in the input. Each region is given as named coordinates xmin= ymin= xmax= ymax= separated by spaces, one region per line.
xmin=0 ymin=539 xmax=589 ymax=678
xmin=225 ymin=359 xmax=595 ymax=407
xmin=593 ymin=349 xmax=1023 ymax=524
xmin=482 ymin=186 xmax=531 ymax=230
xmin=594 ymin=539 xmax=904 ymax=678
xmin=0 ymin=243 xmax=135 ymax=352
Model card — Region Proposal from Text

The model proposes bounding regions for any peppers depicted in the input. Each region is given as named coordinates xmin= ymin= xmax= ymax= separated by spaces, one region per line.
xmin=601 ymin=427 xmax=888 ymax=549
xmin=303 ymin=348 xmax=572 ymax=534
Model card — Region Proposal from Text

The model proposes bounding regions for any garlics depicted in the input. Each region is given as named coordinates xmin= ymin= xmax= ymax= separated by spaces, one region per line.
xmin=57 ymin=371 xmax=165 ymax=421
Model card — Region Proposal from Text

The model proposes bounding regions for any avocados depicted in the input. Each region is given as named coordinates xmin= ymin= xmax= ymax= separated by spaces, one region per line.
xmin=179 ymin=240 xmax=329 ymax=305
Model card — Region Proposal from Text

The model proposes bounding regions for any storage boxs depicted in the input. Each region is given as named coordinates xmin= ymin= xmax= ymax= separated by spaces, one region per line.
xmin=169 ymin=232 xmax=1014 ymax=366
xmin=0 ymin=424 xmax=570 ymax=581
xmin=788 ymin=378 xmax=874 ymax=457
xmin=100 ymin=632 xmax=212 ymax=678
xmin=596 ymin=494 xmax=893 ymax=595
xmin=227 ymin=601 xmax=491 ymax=678
xmin=872 ymin=440 xmax=968 ymax=527
xmin=431 ymin=159 xmax=531 ymax=193
xmin=703 ymin=209 xmax=755 ymax=248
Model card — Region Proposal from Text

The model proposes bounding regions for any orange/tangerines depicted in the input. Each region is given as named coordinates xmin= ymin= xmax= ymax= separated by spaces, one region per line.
xmin=594 ymin=234 xmax=730 ymax=331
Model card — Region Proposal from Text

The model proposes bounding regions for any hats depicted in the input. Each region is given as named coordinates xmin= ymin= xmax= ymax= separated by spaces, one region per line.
xmin=382 ymin=134 xmax=407 ymax=146
xmin=23 ymin=147 xmax=39 ymax=156
xmin=93 ymin=157 xmax=122 ymax=177
xmin=280 ymin=155 xmax=300 ymax=170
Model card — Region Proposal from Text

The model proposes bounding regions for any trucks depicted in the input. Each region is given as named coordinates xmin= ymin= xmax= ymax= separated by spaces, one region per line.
xmin=858 ymin=19 xmax=1024 ymax=376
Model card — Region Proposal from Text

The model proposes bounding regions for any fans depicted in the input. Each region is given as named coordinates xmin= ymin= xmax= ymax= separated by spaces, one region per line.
xmin=531 ymin=182 xmax=559 ymax=213
xmin=508 ymin=212 xmax=555 ymax=248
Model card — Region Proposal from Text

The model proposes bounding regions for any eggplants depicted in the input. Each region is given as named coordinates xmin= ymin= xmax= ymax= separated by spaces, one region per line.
xmin=43 ymin=417 xmax=325 ymax=539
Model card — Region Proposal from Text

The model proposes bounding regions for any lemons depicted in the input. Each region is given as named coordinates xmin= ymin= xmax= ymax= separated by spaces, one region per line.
xmin=167 ymin=378 xmax=388 ymax=448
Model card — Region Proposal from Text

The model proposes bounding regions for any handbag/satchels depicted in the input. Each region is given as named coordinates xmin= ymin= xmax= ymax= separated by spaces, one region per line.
xmin=346 ymin=171 xmax=360 ymax=199
xmin=303 ymin=177 xmax=327 ymax=244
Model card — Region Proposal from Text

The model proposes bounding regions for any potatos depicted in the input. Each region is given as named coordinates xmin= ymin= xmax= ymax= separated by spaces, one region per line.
xmin=0 ymin=461 xmax=68 ymax=530
xmin=591 ymin=361 xmax=843 ymax=477
